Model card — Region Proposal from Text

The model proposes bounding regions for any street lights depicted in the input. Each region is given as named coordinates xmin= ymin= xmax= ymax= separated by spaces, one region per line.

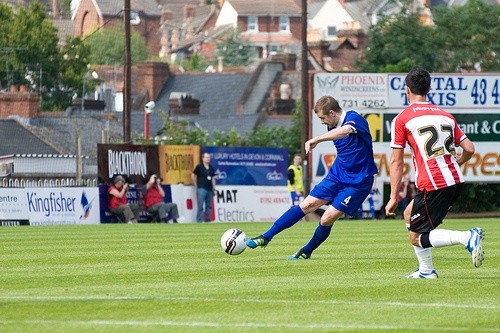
xmin=80 ymin=69 xmax=99 ymax=115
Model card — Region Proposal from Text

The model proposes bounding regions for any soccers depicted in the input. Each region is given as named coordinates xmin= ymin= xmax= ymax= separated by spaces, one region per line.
xmin=220 ymin=228 xmax=248 ymax=256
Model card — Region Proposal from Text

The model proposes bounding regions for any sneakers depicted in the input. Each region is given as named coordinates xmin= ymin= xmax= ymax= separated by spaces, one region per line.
xmin=248 ymin=235 xmax=270 ymax=249
xmin=408 ymin=270 xmax=440 ymax=278
xmin=466 ymin=227 xmax=485 ymax=267
xmin=288 ymin=250 xmax=310 ymax=261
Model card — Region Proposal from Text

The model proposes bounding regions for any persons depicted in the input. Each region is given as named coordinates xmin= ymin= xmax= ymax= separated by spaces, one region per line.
xmin=399 ymin=174 xmax=416 ymax=220
xmin=385 ymin=69 xmax=485 ymax=278
xmin=286 ymin=154 xmax=308 ymax=221
xmin=108 ymin=176 xmax=135 ymax=224
xmin=141 ymin=174 xmax=179 ymax=223
xmin=246 ymin=95 xmax=378 ymax=260
xmin=191 ymin=153 xmax=217 ymax=223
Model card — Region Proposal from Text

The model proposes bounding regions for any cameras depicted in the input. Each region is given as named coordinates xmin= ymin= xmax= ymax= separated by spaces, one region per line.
xmin=154 ymin=177 xmax=163 ymax=185
xmin=121 ymin=183 xmax=137 ymax=189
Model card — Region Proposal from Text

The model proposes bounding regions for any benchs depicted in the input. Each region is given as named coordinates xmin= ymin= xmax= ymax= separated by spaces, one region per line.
xmin=104 ymin=187 xmax=171 ymax=224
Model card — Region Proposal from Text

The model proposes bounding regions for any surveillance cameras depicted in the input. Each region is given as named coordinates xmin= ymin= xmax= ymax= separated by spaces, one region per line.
xmin=144 ymin=100 xmax=155 ymax=113
